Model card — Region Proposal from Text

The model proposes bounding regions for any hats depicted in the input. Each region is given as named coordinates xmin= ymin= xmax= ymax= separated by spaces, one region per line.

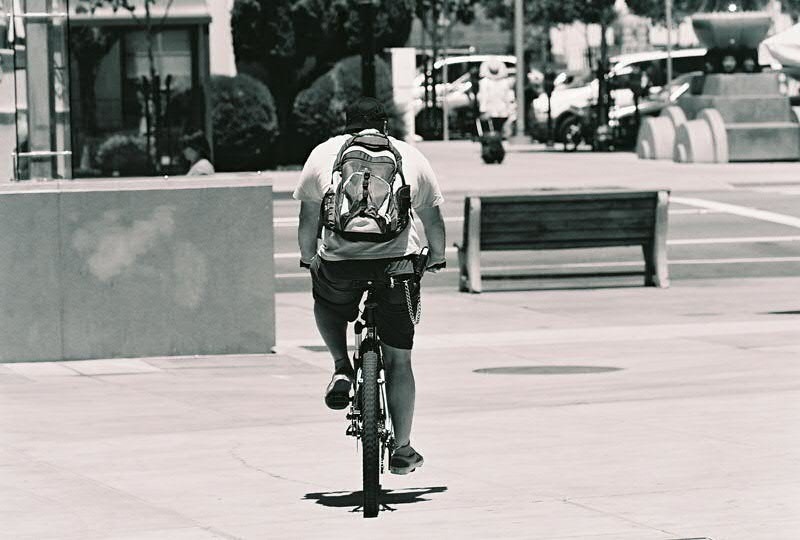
xmin=183 ymin=130 xmax=209 ymax=158
xmin=480 ymin=58 xmax=506 ymax=80
xmin=341 ymin=97 xmax=386 ymax=133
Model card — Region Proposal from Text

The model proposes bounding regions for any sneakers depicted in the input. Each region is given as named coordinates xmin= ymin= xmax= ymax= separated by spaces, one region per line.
xmin=391 ymin=452 xmax=423 ymax=475
xmin=325 ymin=369 xmax=352 ymax=409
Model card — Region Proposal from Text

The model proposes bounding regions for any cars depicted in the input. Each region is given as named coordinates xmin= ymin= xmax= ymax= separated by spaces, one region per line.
xmin=391 ymin=52 xmax=536 ymax=142
xmin=527 ymin=47 xmax=800 ymax=152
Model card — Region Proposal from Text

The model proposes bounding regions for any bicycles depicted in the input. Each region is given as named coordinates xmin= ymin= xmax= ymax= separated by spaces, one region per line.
xmin=298 ymin=255 xmax=448 ymax=519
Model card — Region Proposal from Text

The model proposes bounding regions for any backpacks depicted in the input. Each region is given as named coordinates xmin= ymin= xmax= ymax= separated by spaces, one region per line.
xmin=320 ymin=130 xmax=414 ymax=243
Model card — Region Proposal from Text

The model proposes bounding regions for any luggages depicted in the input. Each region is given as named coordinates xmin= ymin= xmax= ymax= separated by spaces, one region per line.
xmin=476 ymin=117 xmax=504 ymax=164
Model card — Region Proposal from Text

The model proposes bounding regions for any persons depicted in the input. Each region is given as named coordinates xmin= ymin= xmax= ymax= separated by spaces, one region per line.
xmin=182 ymin=143 xmax=214 ymax=176
xmin=478 ymin=57 xmax=510 ymax=136
xmin=292 ymin=96 xmax=446 ymax=473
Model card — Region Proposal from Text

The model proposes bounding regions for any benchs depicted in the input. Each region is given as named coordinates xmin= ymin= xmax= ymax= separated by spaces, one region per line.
xmin=452 ymin=188 xmax=669 ymax=295
